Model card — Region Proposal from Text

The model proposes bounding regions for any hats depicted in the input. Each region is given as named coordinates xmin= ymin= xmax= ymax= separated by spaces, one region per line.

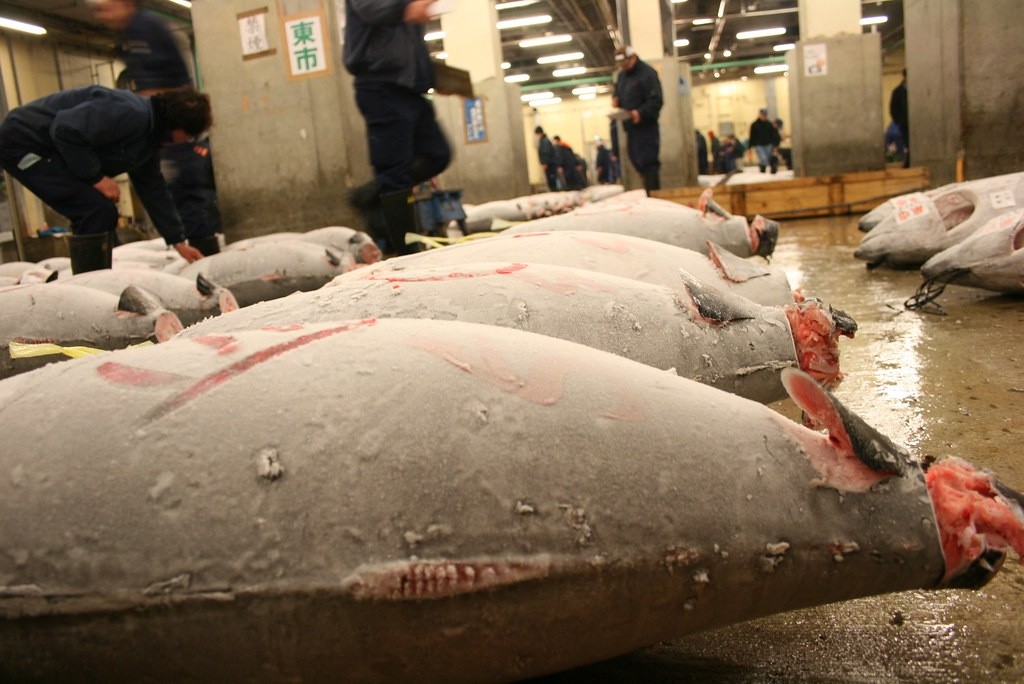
xmin=615 ymin=46 xmax=635 ymax=62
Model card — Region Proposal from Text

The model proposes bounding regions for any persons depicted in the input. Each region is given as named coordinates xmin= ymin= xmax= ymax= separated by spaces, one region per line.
xmin=552 ymin=134 xmax=622 ymax=189
xmin=767 ymin=120 xmax=783 ymax=175
xmin=889 ymin=68 xmax=910 ymax=167
xmin=533 ymin=128 xmax=557 ymax=192
xmin=92 ymin=0 xmax=225 ymax=250
xmin=749 ymin=109 xmax=780 ymax=175
xmin=614 ymin=46 xmax=662 ymax=189
xmin=884 ymin=117 xmax=905 ymax=159
xmin=340 ymin=1 xmax=451 ymax=257
xmin=0 ymin=88 xmax=216 ymax=275
xmin=691 ymin=125 xmax=745 ymax=175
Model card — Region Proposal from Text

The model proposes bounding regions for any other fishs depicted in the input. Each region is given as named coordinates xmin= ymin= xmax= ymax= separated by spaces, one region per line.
xmin=1 ymin=163 xmax=1024 ymax=683
